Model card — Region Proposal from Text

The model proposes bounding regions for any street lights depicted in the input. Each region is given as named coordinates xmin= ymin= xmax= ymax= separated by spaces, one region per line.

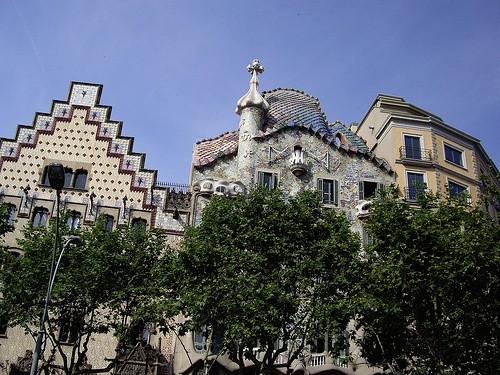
xmin=30 ymin=161 xmax=83 ymax=373
